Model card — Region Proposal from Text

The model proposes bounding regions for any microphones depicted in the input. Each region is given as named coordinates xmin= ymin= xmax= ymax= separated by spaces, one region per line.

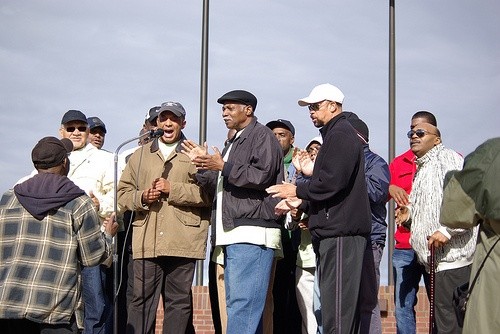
xmin=148 ymin=128 xmax=164 ymax=138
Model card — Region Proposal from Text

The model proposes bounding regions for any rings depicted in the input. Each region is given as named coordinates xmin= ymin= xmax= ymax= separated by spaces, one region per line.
xmin=202 ymin=163 xmax=203 ymax=168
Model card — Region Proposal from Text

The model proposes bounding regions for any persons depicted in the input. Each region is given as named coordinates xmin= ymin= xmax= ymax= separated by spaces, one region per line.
xmin=117 ymin=101 xmax=211 ymax=334
xmin=439 ymin=137 xmax=500 ymax=334
xmin=86 ymin=106 xmax=391 ymax=334
xmin=394 ymin=122 xmax=481 ymax=334
xmin=266 ymin=83 xmax=371 ymax=334
xmin=0 ymin=136 xmax=119 ymax=334
xmin=181 ymin=89 xmax=286 ymax=334
xmin=389 ymin=110 xmax=465 ymax=334
xmin=12 ymin=109 xmax=124 ymax=334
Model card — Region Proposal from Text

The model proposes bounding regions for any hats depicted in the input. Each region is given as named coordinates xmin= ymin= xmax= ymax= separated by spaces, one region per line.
xmin=147 ymin=106 xmax=162 ymax=124
xmin=216 ymin=90 xmax=257 ymax=110
xmin=264 ymin=119 xmax=295 ymax=137
xmin=31 ymin=137 xmax=73 ymax=167
xmin=158 ymin=102 xmax=186 ymax=119
xmin=347 ymin=119 xmax=369 ymax=145
xmin=87 ymin=117 xmax=106 ymax=133
xmin=61 ymin=110 xmax=88 ymax=124
xmin=298 ymin=83 xmax=344 ymax=107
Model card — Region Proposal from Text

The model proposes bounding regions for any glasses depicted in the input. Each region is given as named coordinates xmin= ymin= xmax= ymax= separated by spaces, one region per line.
xmin=309 ymin=101 xmax=331 ymax=111
xmin=407 ymin=130 xmax=438 ymax=138
xmin=60 ymin=126 xmax=88 ymax=132
